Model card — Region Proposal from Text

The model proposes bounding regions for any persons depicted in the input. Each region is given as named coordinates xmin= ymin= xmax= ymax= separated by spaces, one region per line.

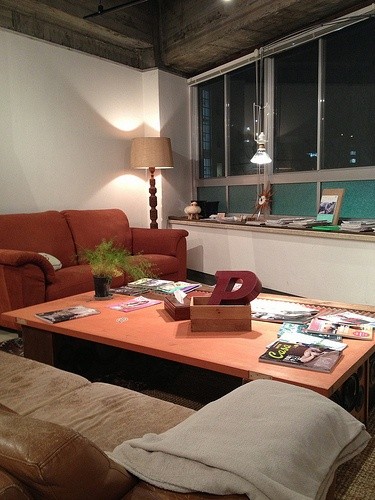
xmin=296 ymin=348 xmax=321 ymax=364
xmin=111 ymin=301 xmax=149 ymax=311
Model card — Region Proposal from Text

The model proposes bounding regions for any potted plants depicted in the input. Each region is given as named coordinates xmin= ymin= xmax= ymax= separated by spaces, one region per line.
xmin=70 ymin=234 xmax=163 ymax=298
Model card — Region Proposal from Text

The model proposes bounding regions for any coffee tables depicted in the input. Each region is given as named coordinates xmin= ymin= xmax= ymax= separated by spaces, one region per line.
xmin=0 ymin=280 xmax=375 ymax=430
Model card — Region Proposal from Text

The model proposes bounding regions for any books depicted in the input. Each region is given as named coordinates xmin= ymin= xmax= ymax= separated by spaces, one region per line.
xmin=277 ymin=320 xmax=343 ymax=343
xmin=107 ymin=296 xmax=162 ymax=313
xmin=250 ymin=311 xmax=375 ymax=341
xmin=34 ymin=305 xmax=100 ymax=324
xmin=266 ymin=332 xmax=348 ymax=352
xmin=266 ymin=216 xmax=333 ymax=229
xmin=108 ymin=277 xmax=202 ymax=296
xmin=258 ymin=341 xmax=343 ymax=373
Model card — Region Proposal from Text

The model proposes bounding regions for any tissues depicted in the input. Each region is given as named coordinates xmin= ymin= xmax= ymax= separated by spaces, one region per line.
xmin=164 ymin=289 xmax=190 ymax=321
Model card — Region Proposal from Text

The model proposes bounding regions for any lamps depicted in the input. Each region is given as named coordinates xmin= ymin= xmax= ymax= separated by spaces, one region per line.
xmin=129 ymin=137 xmax=175 ymax=230
xmin=249 ymin=102 xmax=273 ymax=213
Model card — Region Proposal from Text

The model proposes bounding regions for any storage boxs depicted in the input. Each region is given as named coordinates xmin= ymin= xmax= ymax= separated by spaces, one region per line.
xmin=189 ymin=296 xmax=252 ymax=331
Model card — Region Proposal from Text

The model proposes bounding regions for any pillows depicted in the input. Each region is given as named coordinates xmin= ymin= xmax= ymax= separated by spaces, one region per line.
xmin=38 ymin=252 xmax=63 ymax=272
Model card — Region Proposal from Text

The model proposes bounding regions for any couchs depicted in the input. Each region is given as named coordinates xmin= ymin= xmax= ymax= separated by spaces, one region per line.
xmin=0 ymin=208 xmax=189 ymax=338
xmin=0 ymin=347 xmax=375 ymax=500
